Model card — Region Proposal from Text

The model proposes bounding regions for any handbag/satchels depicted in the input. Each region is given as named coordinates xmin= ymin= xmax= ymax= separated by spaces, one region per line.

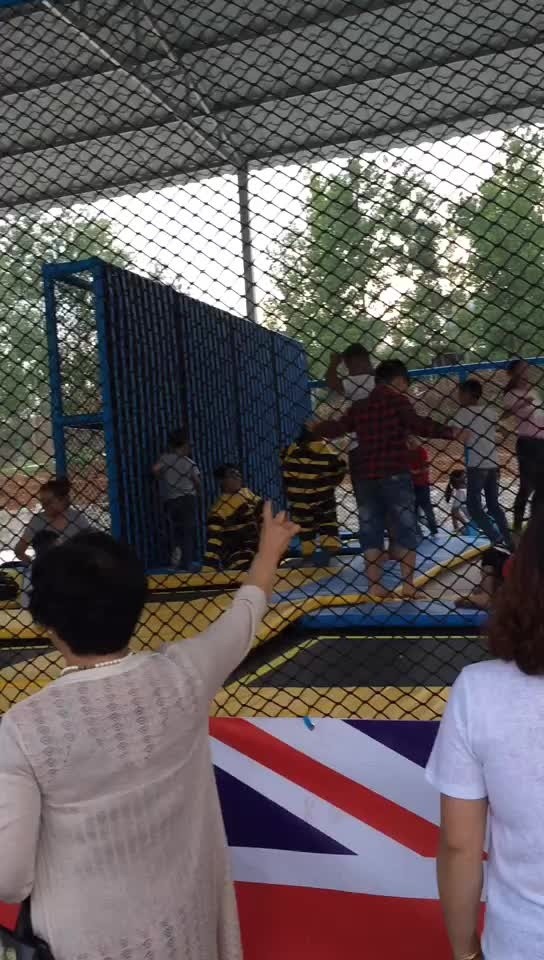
xmin=0 ymin=896 xmax=55 ymax=960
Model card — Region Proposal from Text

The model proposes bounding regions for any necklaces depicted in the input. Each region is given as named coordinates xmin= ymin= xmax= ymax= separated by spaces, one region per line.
xmin=61 ymin=652 xmax=134 ymax=673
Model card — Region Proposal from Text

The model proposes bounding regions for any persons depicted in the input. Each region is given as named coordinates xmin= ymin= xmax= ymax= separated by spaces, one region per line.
xmin=282 ymin=424 xmax=346 ymax=556
xmin=408 ymin=435 xmax=440 ymax=538
xmin=501 ymin=360 xmax=544 ymax=542
xmin=325 ymin=344 xmax=375 ymax=473
xmin=446 ymin=378 xmax=512 ymax=544
xmin=203 ymin=463 xmax=263 ymax=572
xmin=308 ymin=359 xmax=472 ymax=601
xmin=424 ymin=521 xmax=544 ymax=960
xmin=22 ymin=530 xmax=63 ymax=609
xmin=444 ymin=469 xmax=495 ymax=535
xmin=0 ymin=498 xmax=301 ymax=960
xmin=152 ymin=429 xmax=202 ymax=569
xmin=14 ymin=481 xmax=89 ymax=562
